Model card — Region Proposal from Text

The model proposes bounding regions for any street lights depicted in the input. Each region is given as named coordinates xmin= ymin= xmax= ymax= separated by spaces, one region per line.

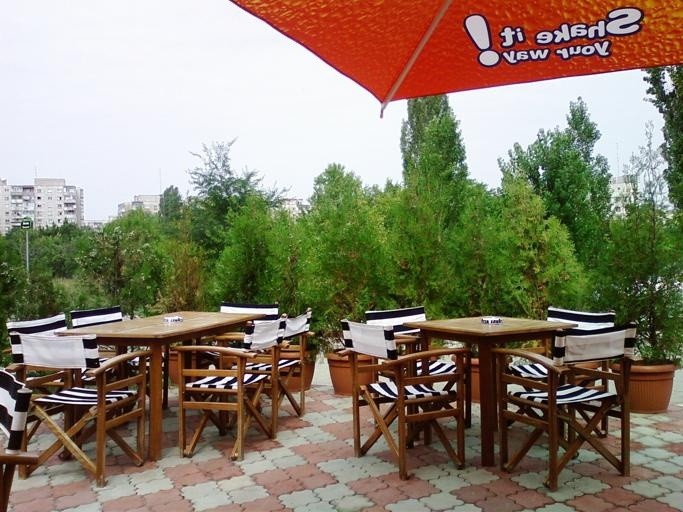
xmin=19 ymin=219 xmax=33 ymax=274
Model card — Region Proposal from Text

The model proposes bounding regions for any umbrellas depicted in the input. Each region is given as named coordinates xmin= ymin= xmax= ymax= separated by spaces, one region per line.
xmin=225 ymin=0 xmax=681 ymax=119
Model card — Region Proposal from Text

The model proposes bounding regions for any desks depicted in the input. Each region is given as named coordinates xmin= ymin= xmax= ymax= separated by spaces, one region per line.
xmin=400 ymin=316 xmax=580 ymax=469
xmin=55 ymin=310 xmax=268 ymax=463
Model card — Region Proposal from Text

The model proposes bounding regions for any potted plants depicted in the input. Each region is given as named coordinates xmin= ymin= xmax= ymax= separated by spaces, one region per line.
xmin=166 ymin=164 xmax=682 ymax=413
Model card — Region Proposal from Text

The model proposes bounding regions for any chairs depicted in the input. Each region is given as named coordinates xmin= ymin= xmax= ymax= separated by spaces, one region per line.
xmin=492 ymin=327 xmax=636 ymax=492
xmin=6 ymin=314 xmax=101 ymax=462
xmin=1 ymin=367 xmax=31 ymax=512
xmin=338 ymin=318 xmax=470 ymax=482
xmin=71 ymin=305 xmax=166 ymax=412
xmin=173 ymin=312 xmax=287 ymax=464
xmin=16 ymin=333 xmax=149 ymax=487
